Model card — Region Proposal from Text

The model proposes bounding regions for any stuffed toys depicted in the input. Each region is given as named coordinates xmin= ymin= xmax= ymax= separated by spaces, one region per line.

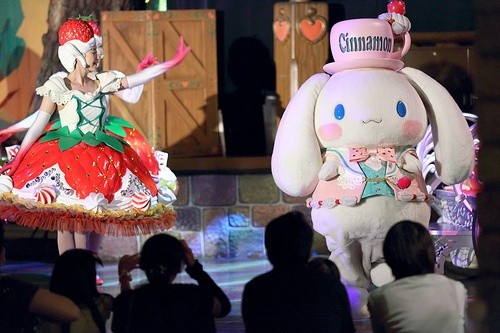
xmin=270 ymin=0 xmax=474 ymax=320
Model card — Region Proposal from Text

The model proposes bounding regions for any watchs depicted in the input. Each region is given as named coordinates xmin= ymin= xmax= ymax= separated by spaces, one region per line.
xmin=118 ymin=270 xmax=133 ymax=283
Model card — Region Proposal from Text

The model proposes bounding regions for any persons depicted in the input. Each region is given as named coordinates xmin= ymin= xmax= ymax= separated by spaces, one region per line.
xmin=0 ymin=14 xmax=192 ymax=285
xmin=367 ymin=219 xmax=468 ymax=333
xmin=241 ymin=211 xmax=357 ymax=333
xmin=0 ymin=232 xmax=231 ymax=333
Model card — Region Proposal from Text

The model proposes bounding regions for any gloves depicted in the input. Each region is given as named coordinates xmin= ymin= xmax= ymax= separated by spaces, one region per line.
xmin=127 ymin=35 xmax=192 ymax=88
xmin=0 ymin=109 xmax=39 ymax=142
xmin=0 ymin=110 xmax=51 ymax=175
xmin=123 ymin=52 xmax=159 ymax=103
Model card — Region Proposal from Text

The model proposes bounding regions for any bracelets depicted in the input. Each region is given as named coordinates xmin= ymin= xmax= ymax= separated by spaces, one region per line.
xmin=185 ymin=259 xmax=202 ymax=278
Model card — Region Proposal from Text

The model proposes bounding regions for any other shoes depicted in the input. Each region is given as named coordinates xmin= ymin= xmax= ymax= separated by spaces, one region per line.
xmin=96 ymin=273 xmax=103 ymax=284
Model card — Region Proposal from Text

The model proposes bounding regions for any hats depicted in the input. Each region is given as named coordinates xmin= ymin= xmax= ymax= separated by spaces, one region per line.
xmin=85 ymin=14 xmax=102 ymax=40
xmin=58 ymin=14 xmax=96 ymax=72
